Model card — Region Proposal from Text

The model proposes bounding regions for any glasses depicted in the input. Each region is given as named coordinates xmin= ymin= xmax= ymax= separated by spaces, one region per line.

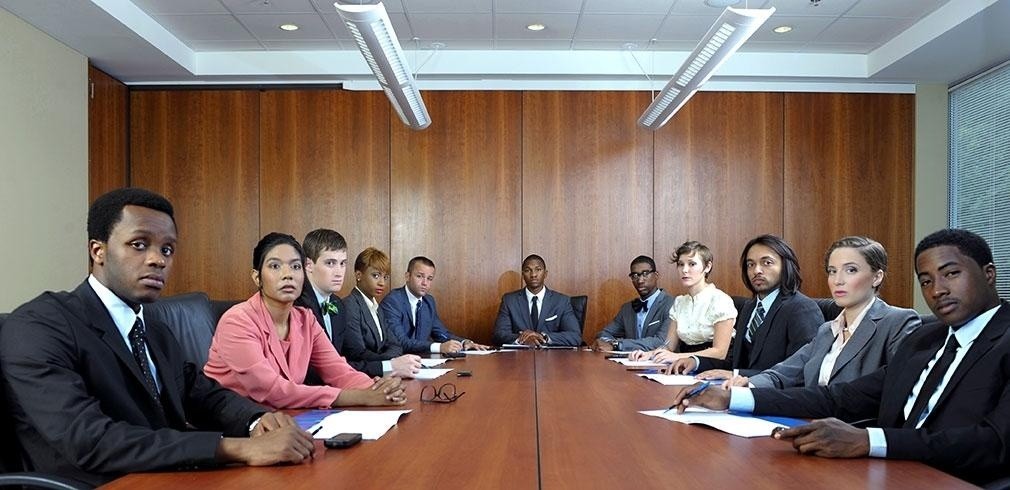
xmin=420 ymin=384 xmax=465 ymax=403
xmin=628 ymin=270 xmax=653 ymax=280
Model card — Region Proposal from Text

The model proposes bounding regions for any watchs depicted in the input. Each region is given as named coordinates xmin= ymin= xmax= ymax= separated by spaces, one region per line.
xmin=611 ymin=339 xmax=618 ymax=350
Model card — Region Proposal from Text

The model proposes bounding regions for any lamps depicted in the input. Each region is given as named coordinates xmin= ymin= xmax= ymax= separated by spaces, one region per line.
xmin=623 ymin=0 xmax=776 ymax=131
xmin=334 ymin=0 xmax=444 ymax=131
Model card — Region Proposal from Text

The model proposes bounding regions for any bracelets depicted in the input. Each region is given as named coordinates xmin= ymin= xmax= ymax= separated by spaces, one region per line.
xmin=541 ymin=331 xmax=549 ymax=343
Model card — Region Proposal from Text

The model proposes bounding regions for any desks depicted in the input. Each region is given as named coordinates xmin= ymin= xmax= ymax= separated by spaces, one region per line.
xmin=96 ymin=348 xmax=539 ymax=490
xmin=534 ymin=345 xmax=984 ymax=490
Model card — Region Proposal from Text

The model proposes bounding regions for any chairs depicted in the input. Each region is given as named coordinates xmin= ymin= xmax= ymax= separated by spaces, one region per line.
xmin=571 ymin=295 xmax=588 ymax=334
xmin=812 ymin=297 xmax=845 ymax=322
xmin=144 ymin=291 xmax=218 ymax=370
xmin=921 ymin=313 xmax=939 ymax=324
xmin=731 ymin=295 xmax=749 ymax=312
xmin=210 ymin=299 xmax=248 ymax=320
xmin=0 ymin=314 xmax=94 ymax=490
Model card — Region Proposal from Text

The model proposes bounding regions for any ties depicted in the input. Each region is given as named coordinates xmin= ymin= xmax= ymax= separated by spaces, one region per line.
xmin=127 ymin=316 xmax=168 ymax=416
xmin=414 ymin=299 xmax=422 ymax=339
xmin=906 ymin=334 xmax=960 ymax=430
xmin=748 ymin=302 xmax=765 ymax=337
xmin=530 ymin=296 xmax=538 ymax=333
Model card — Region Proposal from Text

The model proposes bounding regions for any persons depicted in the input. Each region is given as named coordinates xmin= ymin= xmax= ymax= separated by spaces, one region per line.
xmin=588 ymin=256 xmax=675 ymax=353
xmin=379 ymin=256 xmax=491 ymax=354
xmin=302 ymin=228 xmax=422 ymax=379
xmin=202 ymin=232 xmax=408 ymax=410
xmin=341 ymin=247 xmax=404 ymax=362
xmin=0 ymin=188 xmax=317 ymax=490
xmin=721 ymin=235 xmax=922 ymax=391
xmin=673 ymin=228 xmax=1010 ymax=475
xmin=664 ymin=235 xmax=825 ymax=380
xmin=628 ymin=241 xmax=738 ymax=364
xmin=493 ymin=254 xmax=583 ymax=348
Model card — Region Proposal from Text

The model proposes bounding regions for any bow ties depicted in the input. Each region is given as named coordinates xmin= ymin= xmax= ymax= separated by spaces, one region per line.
xmin=631 ymin=298 xmax=648 ymax=314
xmin=321 ymin=302 xmax=339 ymax=315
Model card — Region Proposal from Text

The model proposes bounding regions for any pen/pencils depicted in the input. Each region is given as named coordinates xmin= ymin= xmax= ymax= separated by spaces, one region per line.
xmin=648 ymin=339 xmax=670 ymax=360
xmin=664 ymin=381 xmax=710 ymax=413
xmin=312 ymin=426 xmax=323 ymax=436
